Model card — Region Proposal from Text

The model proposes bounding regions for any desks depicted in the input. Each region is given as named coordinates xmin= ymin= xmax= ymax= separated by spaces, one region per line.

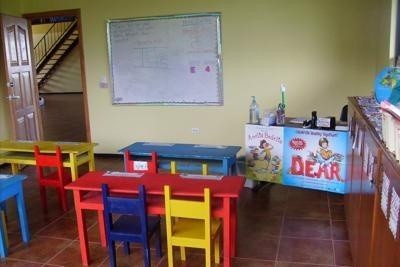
xmin=246 ymin=116 xmax=350 ymax=195
xmin=0 ymin=139 xmax=246 ymax=267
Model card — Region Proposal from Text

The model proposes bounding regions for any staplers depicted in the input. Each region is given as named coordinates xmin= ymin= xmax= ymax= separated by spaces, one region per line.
xmin=305 ymin=119 xmax=311 ymax=125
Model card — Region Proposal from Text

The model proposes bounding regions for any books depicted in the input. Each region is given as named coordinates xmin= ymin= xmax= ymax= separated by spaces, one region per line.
xmin=319 ymin=148 xmax=332 ymax=160
xmin=379 ymin=99 xmax=400 ymax=165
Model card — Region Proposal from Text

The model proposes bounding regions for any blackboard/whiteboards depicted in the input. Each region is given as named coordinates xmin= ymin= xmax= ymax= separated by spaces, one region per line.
xmin=106 ymin=12 xmax=224 ymax=106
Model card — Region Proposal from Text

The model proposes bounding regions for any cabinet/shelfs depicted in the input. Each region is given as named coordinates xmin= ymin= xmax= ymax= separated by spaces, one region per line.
xmin=343 ymin=96 xmax=400 ymax=267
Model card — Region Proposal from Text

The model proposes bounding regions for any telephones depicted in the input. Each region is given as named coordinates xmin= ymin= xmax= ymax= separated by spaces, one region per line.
xmin=312 ymin=111 xmax=316 ymax=127
